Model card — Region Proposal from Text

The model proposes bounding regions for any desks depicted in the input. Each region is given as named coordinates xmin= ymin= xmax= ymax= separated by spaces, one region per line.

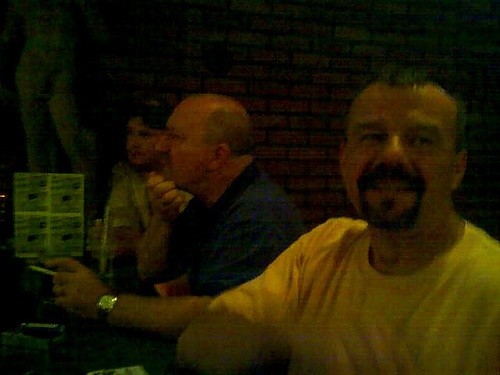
xmin=0 ymin=243 xmax=269 ymax=374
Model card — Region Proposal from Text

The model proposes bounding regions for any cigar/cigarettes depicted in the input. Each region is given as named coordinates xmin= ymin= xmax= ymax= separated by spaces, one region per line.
xmin=27 ymin=265 xmax=56 ymax=277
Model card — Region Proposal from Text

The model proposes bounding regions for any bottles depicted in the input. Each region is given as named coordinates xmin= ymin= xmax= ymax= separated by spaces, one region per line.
xmin=98 ymin=205 xmax=120 ymax=282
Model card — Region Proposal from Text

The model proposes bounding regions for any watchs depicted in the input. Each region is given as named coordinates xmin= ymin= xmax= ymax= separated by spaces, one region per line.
xmin=96 ymin=287 xmax=121 ymax=327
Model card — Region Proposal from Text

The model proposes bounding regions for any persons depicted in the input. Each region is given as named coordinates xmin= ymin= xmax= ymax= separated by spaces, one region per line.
xmin=44 ymin=92 xmax=308 ymax=341
xmin=175 ymin=61 xmax=500 ymax=375
xmin=102 ymin=100 xmax=169 ymax=259
